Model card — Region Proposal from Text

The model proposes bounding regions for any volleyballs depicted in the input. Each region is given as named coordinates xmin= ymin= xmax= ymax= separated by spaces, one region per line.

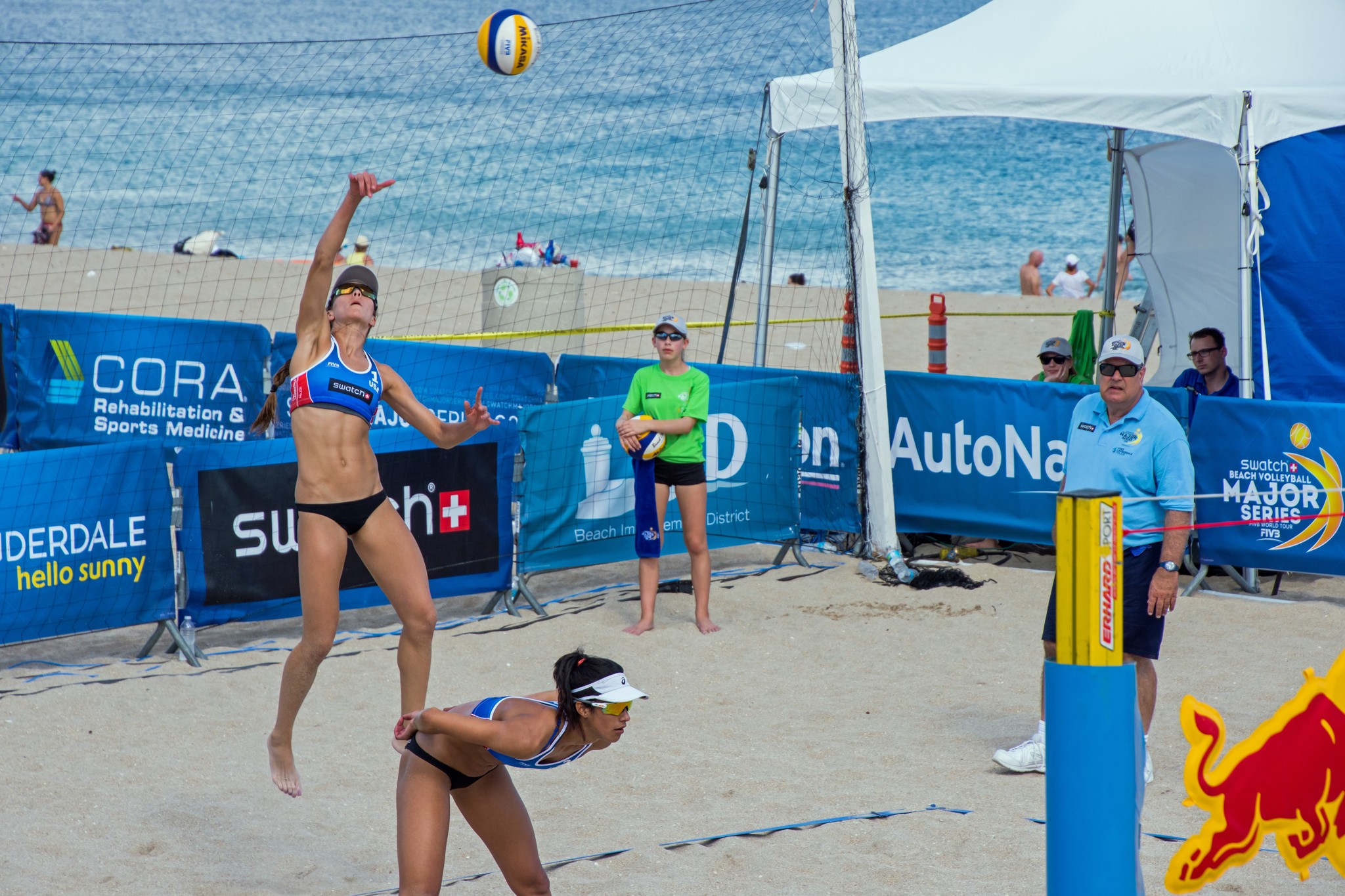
xmin=478 ymin=9 xmax=541 ymax=77
xmin=626 ymin=414 xmax=666 ymax=460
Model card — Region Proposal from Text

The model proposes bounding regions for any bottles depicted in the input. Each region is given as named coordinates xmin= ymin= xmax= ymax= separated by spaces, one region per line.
xmin=898 ymin=569 xmax=919 ymax=583
xmin=179 ymin=615 xmax=195 ymax=660
xmin=858 ymin=560 xmax=878 ymax=579
xmin=886 ymin=546 xmax=909 ymax=574
xmin=801 ymin=542 xmax=837 ymax=554
xmin=494 ymin=577 xmax=520 ymax=612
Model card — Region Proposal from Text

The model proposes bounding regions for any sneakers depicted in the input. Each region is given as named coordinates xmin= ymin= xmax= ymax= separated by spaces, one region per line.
xmin=1144 ymin=750 xmax=1154 ymax=785
xmin=992 ymin=733 xmax=1046 ymax=774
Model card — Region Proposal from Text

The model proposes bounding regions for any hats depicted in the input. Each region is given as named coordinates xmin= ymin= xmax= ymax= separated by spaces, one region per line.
xmin=332 ymin=264 xmax=379 ymax=298
xmin=1037 ymin=337 xmax=1073 ymax=359
xmin=571 ymin=672 xmax=649 ymax=703
xmin=1065 ymin=253 xmax=1080 ymax=266
xmin=653 ymin=313 xmax=688 ymax=339
xmin=355 ymin=235 xmax=369 ymax=248
xmin=1099 ymin=334 xmax=1144 ymax=366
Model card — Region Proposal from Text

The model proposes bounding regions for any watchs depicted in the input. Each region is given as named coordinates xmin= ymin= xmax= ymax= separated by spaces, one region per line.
xmin=1157 ymin=560 xmax=1180 ymax=573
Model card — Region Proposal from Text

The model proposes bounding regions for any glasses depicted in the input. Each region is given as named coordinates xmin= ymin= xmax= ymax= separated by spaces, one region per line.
xmin=1039 ymin=354 xmax=1071 ymax=365
xmin=571 ymin=697 xmax=632 ymax=716
xmin=1099 ymin=362 xmax=1142 ymax=377
xmin=654 ymin=331 xmax=685 ymax=341
xmin=329 ymin=282 xmax=378 ymax=316
xmin=1187 ymin=347 xmax=1221 ymax=360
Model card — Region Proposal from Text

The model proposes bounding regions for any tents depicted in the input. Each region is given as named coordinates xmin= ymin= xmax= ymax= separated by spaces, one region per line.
xmin=752 ymin=1 xmax=1344 ymax=403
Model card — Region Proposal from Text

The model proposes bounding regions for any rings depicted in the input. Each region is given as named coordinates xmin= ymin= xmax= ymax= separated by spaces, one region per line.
xmin=1062 ymin=373 xmax=1065 ymax=377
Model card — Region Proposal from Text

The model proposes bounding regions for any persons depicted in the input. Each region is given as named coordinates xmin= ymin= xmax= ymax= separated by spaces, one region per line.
xmin=394 ymin=649 xmax=650 ymax=896
xmin=332 ymin=235 xmax=377 ymax=264
xmin=13 ymin=171 xmax=66 ymax=245
xmin=992 ymin=197 xmax=1240 ymax=782
xmin=268 ymin=173 xmax=502 ymax=798
xmin=615 ymin=315 xmax=723 ymax=635
